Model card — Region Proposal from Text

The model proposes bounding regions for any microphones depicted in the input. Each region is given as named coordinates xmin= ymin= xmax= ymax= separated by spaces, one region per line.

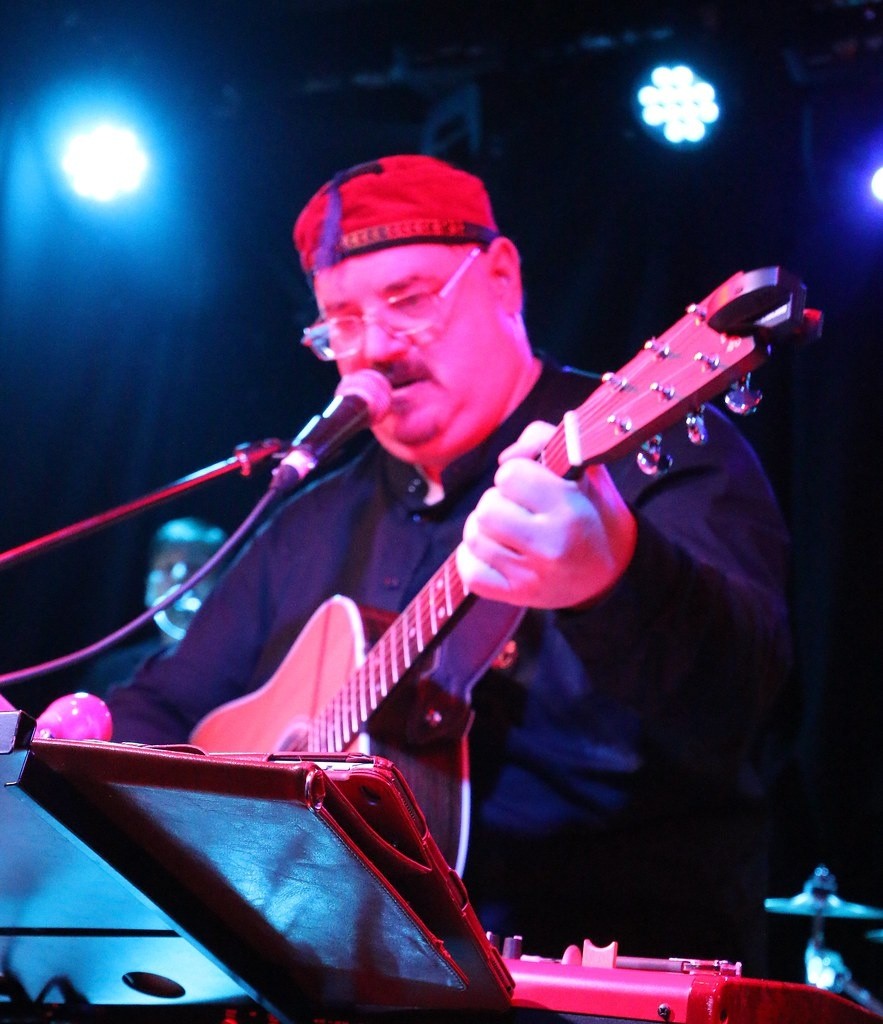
xmin=267 ymin=367 xmax=393 ymax=496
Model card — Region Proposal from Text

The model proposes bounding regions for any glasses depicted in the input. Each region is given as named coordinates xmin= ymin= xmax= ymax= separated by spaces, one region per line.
xmin=300 ymin=249 xmax=480 ymax=361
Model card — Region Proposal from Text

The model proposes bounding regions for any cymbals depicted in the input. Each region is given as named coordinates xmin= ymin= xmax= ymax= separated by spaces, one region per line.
xmin=763 ymin=894 xmax=883 ymax=920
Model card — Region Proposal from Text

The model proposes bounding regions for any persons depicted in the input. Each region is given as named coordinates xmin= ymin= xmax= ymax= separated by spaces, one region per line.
xmin=103 ymin=155 xmax=791 ymax=979
xmin=81 ymin=519 xmax=228 ymax=700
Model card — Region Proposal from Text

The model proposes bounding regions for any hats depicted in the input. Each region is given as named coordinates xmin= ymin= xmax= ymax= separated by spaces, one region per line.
xmin=294 ymin=153 xmax=498 ymax=275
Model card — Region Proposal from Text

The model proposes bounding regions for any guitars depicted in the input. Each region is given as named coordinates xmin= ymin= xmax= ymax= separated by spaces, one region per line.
xmin=187 ymin=262 xmax=830 ymax=878
